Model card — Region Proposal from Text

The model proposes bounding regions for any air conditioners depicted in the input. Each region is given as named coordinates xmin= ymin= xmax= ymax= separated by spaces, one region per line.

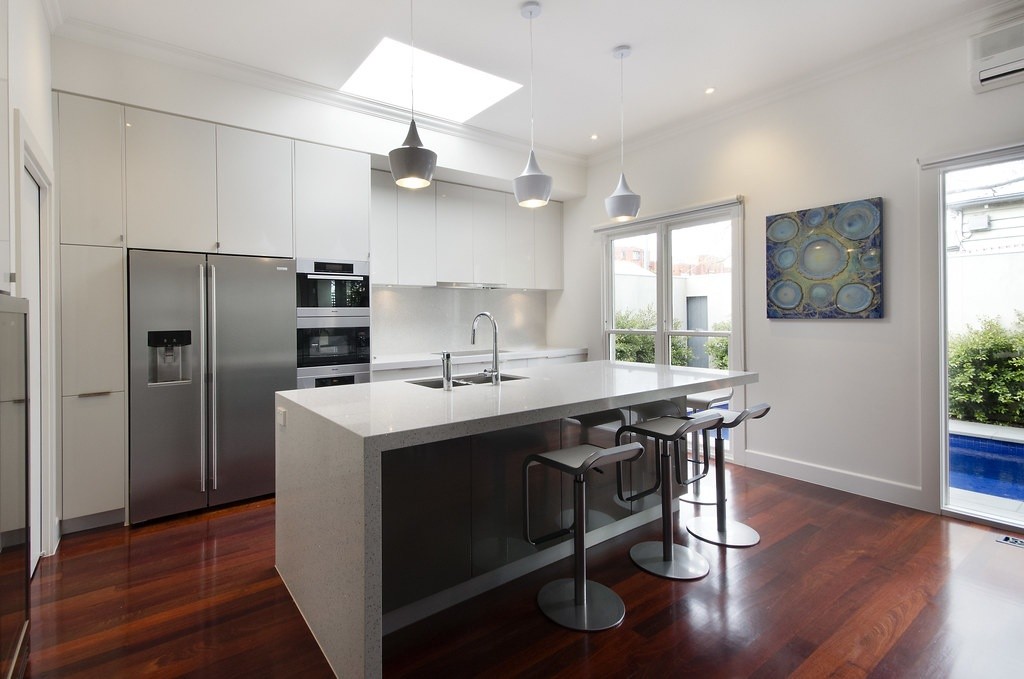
xmin=969 ymin=21 xmax=1024 ymax=95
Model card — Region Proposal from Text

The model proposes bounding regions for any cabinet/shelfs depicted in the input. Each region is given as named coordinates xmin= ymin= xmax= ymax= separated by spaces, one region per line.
xmin=60 ymin=391 xmax=127 ymax=531
xmin=51 ymin=91 xmax=373 ymax=262
xmin=62 ymin=245 xmax=128 ymax=396
xmin=369 ymin=169 xmax=565 ymax=290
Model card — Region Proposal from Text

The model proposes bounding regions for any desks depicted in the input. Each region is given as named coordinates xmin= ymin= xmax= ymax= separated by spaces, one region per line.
xmin=275 ymin=360 xmax=759 ymax=679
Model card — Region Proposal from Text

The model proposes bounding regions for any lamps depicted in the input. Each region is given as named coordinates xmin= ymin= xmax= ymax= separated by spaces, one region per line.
xmin=513 ymin=1 xmax=555 ymax=208
xmin=604 ymin=46 xmax=642 ymax=223
xmin=389 ymin=1 xmax=438 ymax=189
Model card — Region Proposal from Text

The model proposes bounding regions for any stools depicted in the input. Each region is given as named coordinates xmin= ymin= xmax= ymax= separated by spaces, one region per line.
xmin=522 ymin=440 xmax=645 ymax=633
xmin=678 ymin=368 xmax=734 ymax=504
xmin=615 ymin=414 xmax=725 ymax=581
xmin=675 ymin=405 xmax=771 ymax=548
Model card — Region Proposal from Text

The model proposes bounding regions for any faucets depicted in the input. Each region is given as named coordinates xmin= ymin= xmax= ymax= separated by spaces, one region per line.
xmin=441 ymin=351 xmax=453 ymax=391
xmin=471 ymin=311 xmax=501 ymax=386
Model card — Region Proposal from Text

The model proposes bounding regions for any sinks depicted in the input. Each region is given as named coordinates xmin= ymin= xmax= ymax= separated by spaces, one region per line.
xmin=403 ymin=377 xmax=474 ymax=389
xmin=451 ymin=371 xmax=531 ymax=384
